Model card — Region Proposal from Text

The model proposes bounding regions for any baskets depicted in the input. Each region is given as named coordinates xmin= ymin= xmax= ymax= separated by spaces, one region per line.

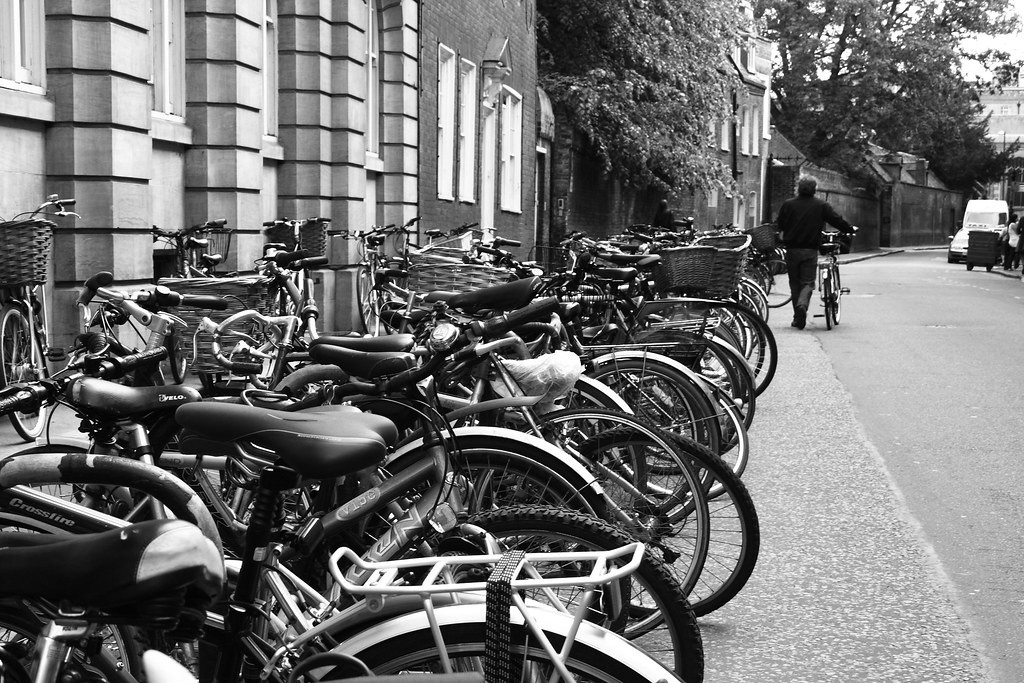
xmin=182 ymin=228 xmax=232 ymax=264
xmin=423 ymin=230 xmax=473 ymax=250
xmin=819 ymin=229 xmax=851 ymax=254
xmin=157 ymin=275 xmax=280 ymax=373
xmin=469 ymin=227 xmax=493 ymax=245
xmin=743 ymin=223 xmax=780 ymax=252
xmin=528 ymin=246 xmax=571 ymax=272
xmin=263 ymin=219 xmax=326 ymax=257
xmin=691 ymin=234 xmax=752 ymax=294
xmin=407 ymin=262 xmax=514 ymax=303
xmin=410 ymin=246 xmax=469 ymax=266
xmin=659 ymin=245 xmax=719 ymax=292
xmin=0 ymin=217 xmax=58 ymax=286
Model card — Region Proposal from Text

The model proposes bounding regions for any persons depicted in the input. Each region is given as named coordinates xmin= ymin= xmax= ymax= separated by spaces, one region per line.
xmin=777 ymin=176 xmax=858 ymax=330
xmin=999 ymin=214 xmax=1022 ymax=271
xmin=1016 ymin=216 xmax=1024 ymax=275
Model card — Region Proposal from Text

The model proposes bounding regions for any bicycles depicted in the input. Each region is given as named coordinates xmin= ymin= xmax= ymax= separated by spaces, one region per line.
xmin=0 ymin=195 xmax=82 ymax=441
xmin=1 ymin=214 xmax=793 ymax=683
xmin=813 ymin=227 xmax=858 ymax=330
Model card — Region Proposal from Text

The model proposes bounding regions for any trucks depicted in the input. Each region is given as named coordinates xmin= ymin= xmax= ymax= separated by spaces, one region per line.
xmin=957 ymin=198 xmax=1010 ymax=227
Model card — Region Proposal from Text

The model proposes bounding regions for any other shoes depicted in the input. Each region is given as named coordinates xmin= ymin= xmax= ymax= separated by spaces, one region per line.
xmin=791 ymin=318 xmax=797 ymax=328
xmin=795 ymin=304 xmax=807 ymax=329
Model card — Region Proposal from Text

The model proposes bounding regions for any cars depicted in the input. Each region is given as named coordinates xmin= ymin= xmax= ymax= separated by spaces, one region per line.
xmin=947 ymin=226 xmax=996 ymax=264
